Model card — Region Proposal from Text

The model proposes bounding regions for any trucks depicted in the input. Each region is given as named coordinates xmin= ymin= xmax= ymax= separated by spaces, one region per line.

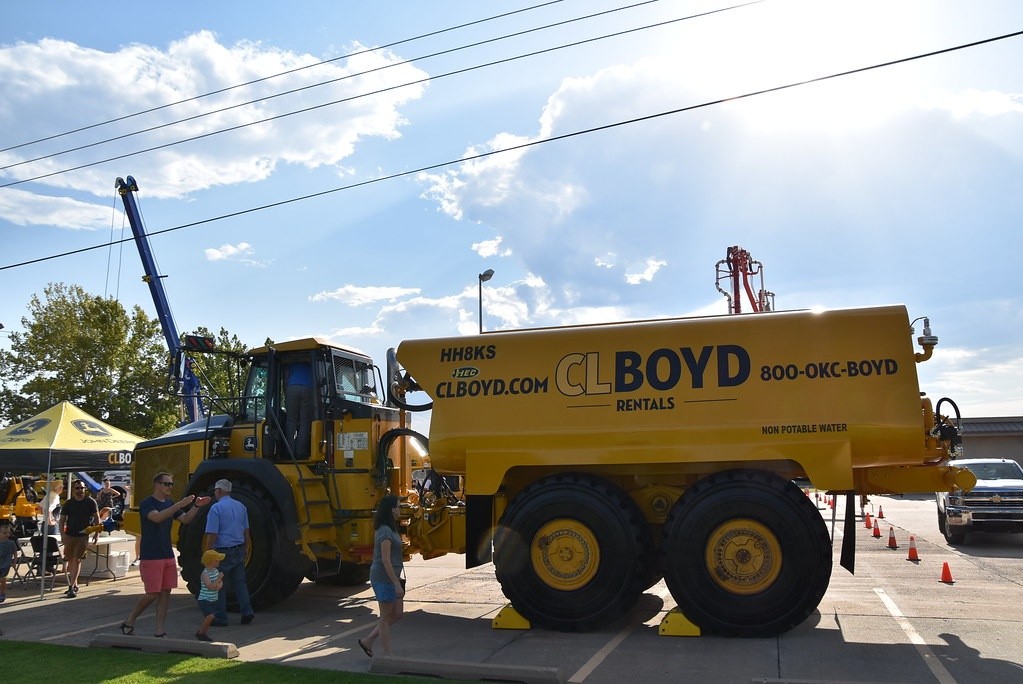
xmin=122 ymin=303 xmax=978 ymax=643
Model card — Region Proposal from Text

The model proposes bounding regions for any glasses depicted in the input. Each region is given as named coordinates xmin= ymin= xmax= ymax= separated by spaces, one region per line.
xmin=157 ymin=482 xmax=174 ymax=487
xmin=74 ymin=487 xmax=84 ymax=490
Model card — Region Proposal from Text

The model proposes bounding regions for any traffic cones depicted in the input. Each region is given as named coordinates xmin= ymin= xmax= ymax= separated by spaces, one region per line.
xmin=863 ymin=512 xmax=875 ymax=528
xmin=876 ymin=505 xmax=886 ymax=518
xmin=818 ymin=493 xmax=834 ymax=509
xmin=871 ymin=519 xmax=883 ymax=537
xmin=886 ymin=526 xmax=899 ymax=548
xmin=938 ymin=561 xmax=957 ymax=583
xmin=905 ymin=536 xmax=922 ymax=561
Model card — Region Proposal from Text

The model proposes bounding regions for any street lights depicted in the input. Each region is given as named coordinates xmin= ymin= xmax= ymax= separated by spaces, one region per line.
xmin=477 ymin=267 xmax=497 ymax=331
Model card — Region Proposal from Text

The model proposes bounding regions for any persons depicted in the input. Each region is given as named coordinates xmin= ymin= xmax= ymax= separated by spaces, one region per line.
xmin=131 ymin=537 xmax=140 ymax=566
xmin=97 ymin=481 xmax=130 ymax=531
xmin=282 ymin=363 xmax=313 ymax=458
xmin=202 ymin=479 xmax=255 ymax=627
xmin=195 ymin=550 xmax=225 ymax=642
xmin=0 ymin=525 xmax=18 ymax=602
xmin=358 ymin=495 xmax=405 ymax=657
xmin=119 ymin=472 xmax=211 ymax=638
xmin=36 ymin=479 xmax=63 ymax=577
xmin=59 ymin=479 xmax=100 ymax=598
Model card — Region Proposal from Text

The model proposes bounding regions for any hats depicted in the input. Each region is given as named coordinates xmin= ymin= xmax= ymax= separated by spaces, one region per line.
xmin=201 ymin=550 xmax=225 ymax=565
xmin=52 ymin=480 xmax=65 ymax=490
xmin=212 ymin=479 xmax=232 ymax=492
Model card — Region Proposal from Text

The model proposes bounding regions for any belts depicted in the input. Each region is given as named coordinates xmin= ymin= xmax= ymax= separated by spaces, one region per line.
xmin=215 ymin=543 xmax=244 ymax=551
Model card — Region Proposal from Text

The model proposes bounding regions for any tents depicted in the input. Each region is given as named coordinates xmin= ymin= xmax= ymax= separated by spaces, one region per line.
xmin=0 ymin=400 xmax=150 ymax=602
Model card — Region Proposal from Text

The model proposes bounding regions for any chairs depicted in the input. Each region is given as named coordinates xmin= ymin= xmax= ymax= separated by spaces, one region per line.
xmin=996 ymin=469 xmax=1010 ymax=478
xmin=25 ymin=536 xmax=71 ymax=591
xmin=8 ymin=536 xmax=38 ymax=589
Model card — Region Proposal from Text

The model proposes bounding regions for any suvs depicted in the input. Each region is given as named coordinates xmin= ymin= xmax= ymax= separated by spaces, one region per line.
xmin=933 ymin=458 xmax=1023 ymax=546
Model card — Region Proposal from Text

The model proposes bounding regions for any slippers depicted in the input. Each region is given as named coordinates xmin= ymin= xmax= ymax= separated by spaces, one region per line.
xmin=358 ymin=639 xmax=373 ymax=658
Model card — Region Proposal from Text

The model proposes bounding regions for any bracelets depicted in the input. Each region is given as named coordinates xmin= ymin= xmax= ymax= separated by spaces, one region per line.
xmin=194 ymin=503 xmax=200 ymax=508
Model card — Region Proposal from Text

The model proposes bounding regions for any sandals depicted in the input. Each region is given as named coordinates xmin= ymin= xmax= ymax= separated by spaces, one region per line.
xmin=153 ymin=632 xmax=168 ymax=639
xmin=119 ymin=619 xmax=135 ymax=636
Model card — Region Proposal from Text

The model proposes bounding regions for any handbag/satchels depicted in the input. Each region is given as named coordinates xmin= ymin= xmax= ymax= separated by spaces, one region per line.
xmin=396 ymin=579 xmax=406 ymax=599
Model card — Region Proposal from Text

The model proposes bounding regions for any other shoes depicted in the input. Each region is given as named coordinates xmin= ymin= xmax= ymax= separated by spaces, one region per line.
xmin=240 ymin=614 xmax=254 ymax=624
xmin=64 ymin=585 xmax=78 ymax=598
xmin=0 ymin=594 xmax=5 ymax=603
xmin=195 ymin=630 xmax=213 ymax=642
xmin=209 ymin=619 xmax=228 ymax=626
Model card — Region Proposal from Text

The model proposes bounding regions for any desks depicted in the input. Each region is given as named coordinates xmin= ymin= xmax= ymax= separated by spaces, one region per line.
xmin=18 ymin=534 xmax=128 ymax=586
